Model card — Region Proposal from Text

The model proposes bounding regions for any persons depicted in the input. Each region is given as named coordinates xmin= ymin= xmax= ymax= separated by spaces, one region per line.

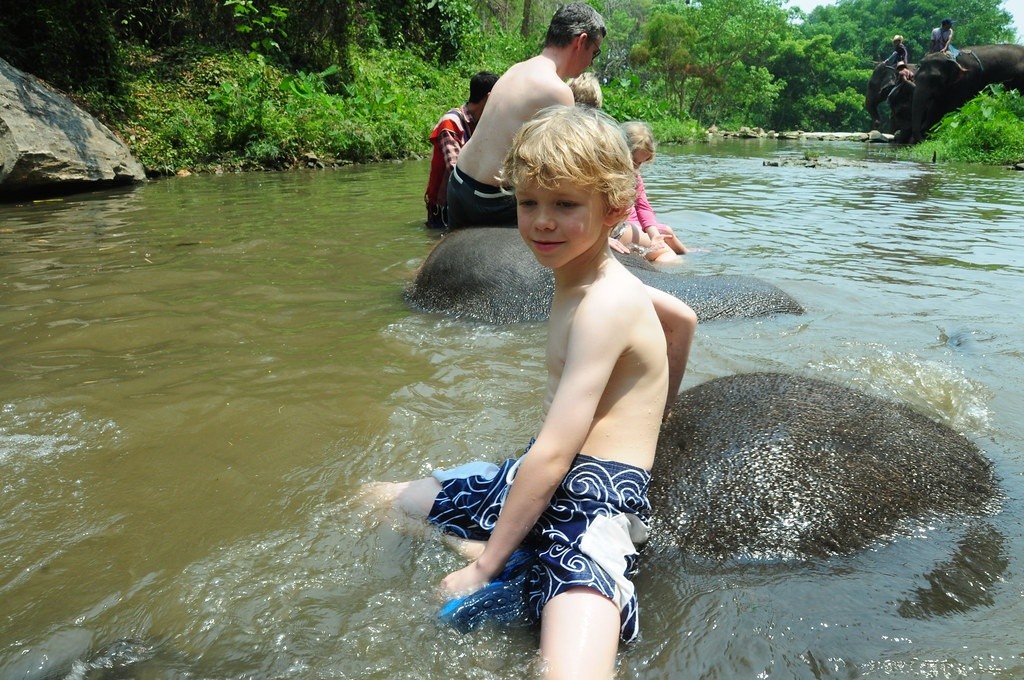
xmin=395 ymin=102 xmax=697 ymax=680
xmin=884 ymin=34 xmax=908 ymax=69
xmin=896 ymin=61 xmax=914 ymax=86
xmin=924 ymin=18 xmax=967 ymax=72
xmin=424 ymin=1 xmax=688 ymax=261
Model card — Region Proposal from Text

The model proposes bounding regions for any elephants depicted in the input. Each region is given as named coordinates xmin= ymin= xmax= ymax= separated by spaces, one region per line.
xmin=414 ymin=227 xmax=996 ymax=558
xmin=865 ymin=41 xmax=1024 ymax=146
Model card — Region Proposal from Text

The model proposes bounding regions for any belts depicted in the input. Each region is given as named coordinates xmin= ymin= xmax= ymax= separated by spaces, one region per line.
xmin=452 ymin=165 xmax=516 ymax=198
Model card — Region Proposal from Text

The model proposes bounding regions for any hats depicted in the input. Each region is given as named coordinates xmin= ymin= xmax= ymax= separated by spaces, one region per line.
xmin=942 ymin=19 xmax=956 ymax=24
xmin=890 ymin=35 xmax=903 ymax=42
xmin=896 ymin=61 xmax=908 ymax=69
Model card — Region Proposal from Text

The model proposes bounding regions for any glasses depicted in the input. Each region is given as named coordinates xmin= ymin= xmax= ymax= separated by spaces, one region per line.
xmin=578 ymin=31 xmax=601 ymax=60
